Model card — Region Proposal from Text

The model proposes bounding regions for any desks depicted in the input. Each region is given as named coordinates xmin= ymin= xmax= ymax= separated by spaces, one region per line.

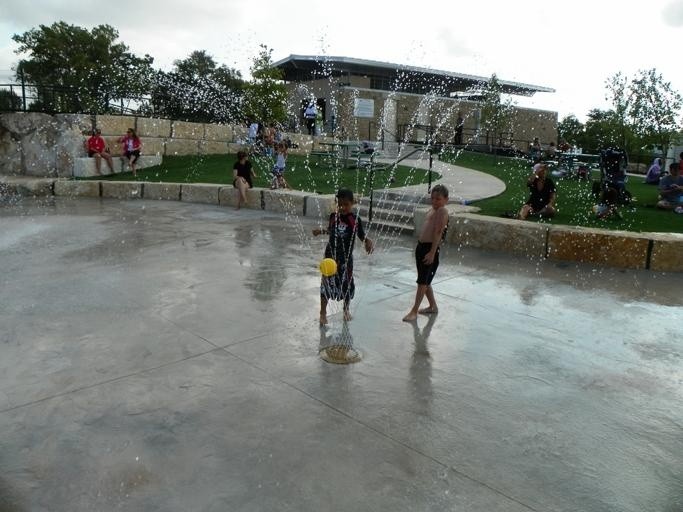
xmin=319 ymin=142 xmax=358 ymax=168
xmin=561 ymin=152 xmax=601 ymax=180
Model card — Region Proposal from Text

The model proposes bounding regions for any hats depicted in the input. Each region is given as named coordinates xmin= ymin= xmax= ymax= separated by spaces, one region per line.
xmin=534 ymin=164 xmax=546 ymax=172
xmin=92 ymin=129 xmax=101 ymax=136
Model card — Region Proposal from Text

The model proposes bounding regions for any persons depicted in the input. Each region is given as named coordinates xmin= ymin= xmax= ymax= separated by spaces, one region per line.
xmin=532 ymin=138 xmax=545 ymax=161
xmin=656 ymin=163 xmax=683 ymax=210
xmin=518 ymin=163 xmax=557 ymax=220
xmin=87 ymin=128 xmax=116 ymax=176
xmin=402 ymin=185 xmax=450 ymax=320
xmin=455 ymin=117 xmax=465 ymax=144
xmin=232 ymin=151 xmax=258 ymax=211
xmin=679 ymin=152 xmax=683 ymax=175
xmin=647 ymin=156 xmax=664 ymax=183
xmin=118 ymin=128 xmax=143 ymax=176
xmin=250 ymin=122 xmax=295 ymax=189
xmin=548 ymin=142 xmax=557 ymax=158
xmin=313 ymin=188 xmax=374 ymax=323
xmin=305 ymin=100 xmax=318 ymax=135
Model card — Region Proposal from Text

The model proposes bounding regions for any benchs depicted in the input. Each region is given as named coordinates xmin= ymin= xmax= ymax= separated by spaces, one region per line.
xmin=73 ymin=155 xmax=162 ymax=177
xmin=311 ymin=149 xmax=378 ymax=170
